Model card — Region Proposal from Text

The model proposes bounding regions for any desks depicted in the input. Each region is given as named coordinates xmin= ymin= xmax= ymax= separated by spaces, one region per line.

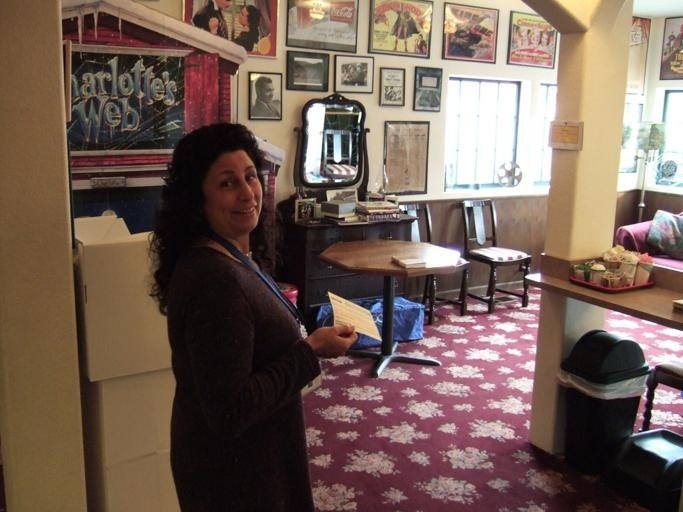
xmin=318 ymin=240 xmax=460 ymax=378
xmin=277 ymin=201 xmax=418 ymax=311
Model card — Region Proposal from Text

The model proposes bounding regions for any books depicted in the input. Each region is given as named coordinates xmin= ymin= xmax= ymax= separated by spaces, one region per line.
xmin=392 ymin=252 xmax=426 ymax=269
xmin=322 ymin=200 xmax=401 ymax=225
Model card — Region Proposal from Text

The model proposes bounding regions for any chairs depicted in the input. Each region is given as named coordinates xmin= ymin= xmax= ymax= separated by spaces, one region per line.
xmin=460 ymin=199 xmax=531 ymax=313
xmin=638 ymin=361 xmax=682 ymax=433
xmin=402 ymin=204 xmax=469 ymax=323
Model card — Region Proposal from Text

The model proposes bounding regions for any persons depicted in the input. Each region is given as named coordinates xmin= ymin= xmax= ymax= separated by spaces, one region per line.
xmin=230 ymin=0 xmax=261 ymax=52
xmin=194 ymin=1 xmax=232 ymax=41
xmin=251 ymin=77 xmax=280 ymax=117
xmin=391 ymin=1 xmax=416 ymax=52
xmin=148 ymin=120 xmax=358 ymax=512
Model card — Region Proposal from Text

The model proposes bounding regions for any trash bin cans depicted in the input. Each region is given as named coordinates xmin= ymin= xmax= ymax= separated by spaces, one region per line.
xmin=559 ymin=330 xmax=654 ymax=476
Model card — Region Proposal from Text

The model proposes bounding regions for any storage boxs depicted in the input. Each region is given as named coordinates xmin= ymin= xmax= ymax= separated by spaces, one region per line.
xmin=84 ymin=445 xmax=182 ymax=510
xmin=73 ymin=215 xmax=174 ymax=385
xmin=82 ymin=369 xmax=176 ymax=467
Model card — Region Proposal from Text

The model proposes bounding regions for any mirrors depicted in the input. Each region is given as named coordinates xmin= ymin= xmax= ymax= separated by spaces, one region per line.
xmin=294 ymin=93 xmax=371 ymax=198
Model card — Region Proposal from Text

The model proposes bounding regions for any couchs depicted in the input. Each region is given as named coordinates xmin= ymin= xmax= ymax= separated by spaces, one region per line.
xmin=616 ymin=212 xmax=682 ymax=272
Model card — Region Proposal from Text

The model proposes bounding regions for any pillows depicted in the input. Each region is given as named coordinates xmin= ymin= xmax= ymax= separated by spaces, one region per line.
xmin=648 ymin=209 xmax=682 ymax=259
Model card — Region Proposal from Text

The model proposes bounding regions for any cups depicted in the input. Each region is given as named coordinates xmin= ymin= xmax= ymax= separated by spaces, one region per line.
xmin=575 ymin=265 xmax=584 ymax=281
xmin=605 ymin=259 xmax=621 ymax=271
xmin=591 ymin=267 xmax=605 ymax=285
xmin=619 ymin=261 xmax=638 ymax=286
xmin=635 ymin=262 xmax=650 ymax=286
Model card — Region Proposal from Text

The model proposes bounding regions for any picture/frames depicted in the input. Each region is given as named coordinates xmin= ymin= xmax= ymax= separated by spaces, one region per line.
xmin=248 ymin=72 xmax=282 ymax=121
xmin=507 ymin=10 xmax=557 ymax=69
xmin=368 ymin=0 xmax=433 ymax=59
xmin=334 ymin=55 xmax=373 ymax=93
xmin=286 ymin=0 xmax=359 ymax=54
xmin=626 ymin=13 xmax=651 ymax=96
xmin=379 ymin=67 xmax=405 ymax=106
xmin=295 ymin=198 xmax=315 ymax=221
xmin=286 ymin=50 xmax=328 ymax=92
xmin=442 ymin=2 xmax=499 ymax=64
xmin=383 ymin=120 xmax=430 ymax=195
xmin=413 ymin=67 xmax=442 ymax=112
xmin=659 ymin=15 xmax=683 ymax=80
xmin=181 ymin=0 xmax=279 ymax=60
